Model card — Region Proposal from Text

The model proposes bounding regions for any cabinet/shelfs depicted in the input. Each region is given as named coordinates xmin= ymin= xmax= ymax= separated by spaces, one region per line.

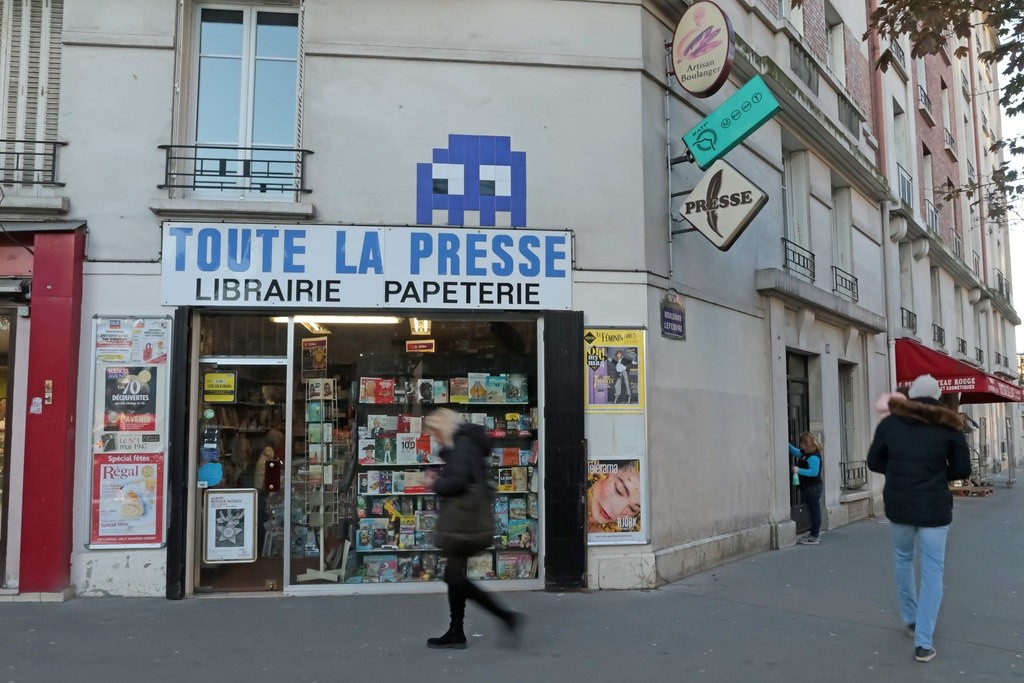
xmin=203 ymin=374 xmax=286 ymax=458
xmin=350 ymin=400 xmax=532 ymax=553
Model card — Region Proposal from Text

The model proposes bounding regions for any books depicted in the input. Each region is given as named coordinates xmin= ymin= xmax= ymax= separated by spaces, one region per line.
xmin=358 ymin=372 xmax=539 ymax=582
xmin=307 ymin=379 xmax=333 ymax=484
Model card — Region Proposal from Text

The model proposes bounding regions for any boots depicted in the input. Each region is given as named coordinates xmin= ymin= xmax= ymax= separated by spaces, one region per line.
xmin=427 ymin=620 xmax=468 ymax=650
xmin=501 ymin=610 xmax=527 ymax=648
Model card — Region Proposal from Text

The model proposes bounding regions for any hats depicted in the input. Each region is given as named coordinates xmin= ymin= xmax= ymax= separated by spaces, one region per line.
xmin=361 ymin=444 xmax=377 ymax=450
xmin=422 ymin=407 xmax=466 ymax=449
xmin=907 ymin=373 xmax=943 ymax=401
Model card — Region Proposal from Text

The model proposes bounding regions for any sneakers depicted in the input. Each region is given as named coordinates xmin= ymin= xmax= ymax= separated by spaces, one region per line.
xmin=799 ymin=534 xmax=821 ymax=545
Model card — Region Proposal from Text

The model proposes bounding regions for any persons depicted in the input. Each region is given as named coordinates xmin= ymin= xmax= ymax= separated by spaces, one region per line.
xmin=423 ymin=408 xmax=524 ymax=649
xmin=406 ymin=382 xmax=417 ymax=403
xmin=324 ymin=383 xmax=332 ymax=397
xmin=371 ymin=418 xmax=385 ymax=438
xmin=420 ymin=382 xmax=434 ymax=403
xmin=789 ymin=432 xmax=823 ymax=545
xmin=866 ymin=373 xmax=972 ymax=662
xmin=608 ymin=350 xmax=638 ymax=403
xmin=586 ymin=465 xmax=640 ymax=533
xmin=310 ymin=384 xmax=320 ymax=398
xmin=238 ymin=430 xmax=284 ymax=549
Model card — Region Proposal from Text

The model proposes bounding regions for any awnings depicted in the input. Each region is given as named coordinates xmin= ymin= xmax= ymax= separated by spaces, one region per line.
xmin=896 ymin=339 xmax=1024 ymax=405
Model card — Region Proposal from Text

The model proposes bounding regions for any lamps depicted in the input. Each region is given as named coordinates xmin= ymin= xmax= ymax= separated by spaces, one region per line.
xmin=408 ymin=318 xmax=431 ymax=336
xmin=301 ymin=322 xmax=334 ymax=334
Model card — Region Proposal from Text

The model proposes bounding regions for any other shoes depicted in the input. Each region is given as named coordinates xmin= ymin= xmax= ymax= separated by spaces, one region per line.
xmin=903 ymin=623 xmax=915 ymax=637
xmin=914 ymin=645 xmax=937 ymax=662
